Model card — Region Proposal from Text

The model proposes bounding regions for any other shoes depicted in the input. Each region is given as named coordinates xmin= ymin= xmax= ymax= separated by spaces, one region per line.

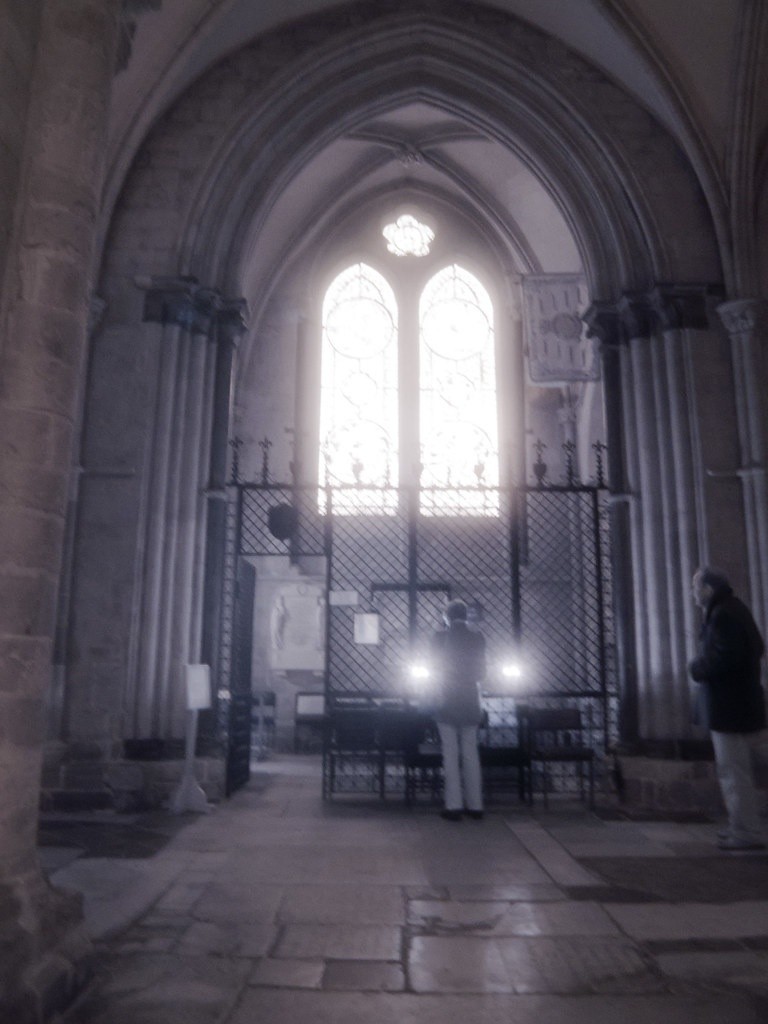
xmin=720 ymin=829 xmax=761 ymax=851
xmin=438 ymin=809 xmax=484 ymax=822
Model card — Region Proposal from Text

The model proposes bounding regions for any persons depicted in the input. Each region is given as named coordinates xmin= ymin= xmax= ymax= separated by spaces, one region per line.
xmin=419 ymin=600 xmax=487 ymax=821
xmin=689 ymin=566 xmax=768 ymax=850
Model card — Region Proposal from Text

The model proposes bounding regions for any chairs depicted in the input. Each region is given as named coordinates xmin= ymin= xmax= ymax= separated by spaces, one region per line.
xmin=317 ymin=700 xmax=599 ymax=810
